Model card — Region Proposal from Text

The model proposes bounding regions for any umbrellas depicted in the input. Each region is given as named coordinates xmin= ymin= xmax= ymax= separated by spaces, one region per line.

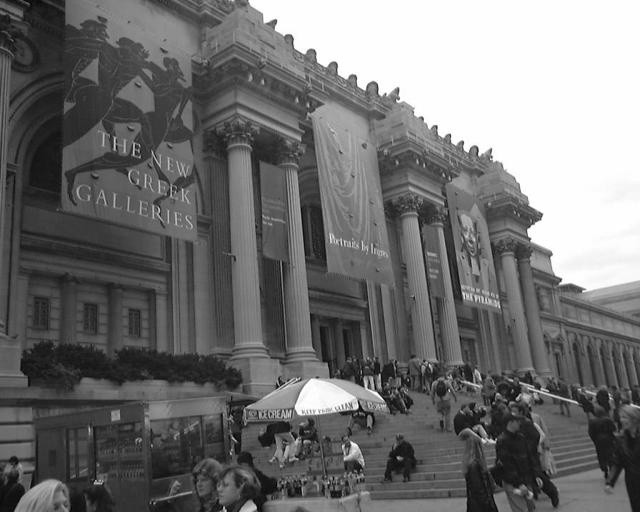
xmin=243 ymin=376 xmax=391 ymax=479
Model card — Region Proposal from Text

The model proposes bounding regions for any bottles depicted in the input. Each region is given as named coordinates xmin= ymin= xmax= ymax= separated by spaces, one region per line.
xmin=274 ymin=468 xmax=366 ymax=501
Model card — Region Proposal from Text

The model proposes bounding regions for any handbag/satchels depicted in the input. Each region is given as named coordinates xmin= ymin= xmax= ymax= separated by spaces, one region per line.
xmin=489 ymin=463 xmax=507 ymax=487
xmin=540 ymin=450 xmax=557 ymax=479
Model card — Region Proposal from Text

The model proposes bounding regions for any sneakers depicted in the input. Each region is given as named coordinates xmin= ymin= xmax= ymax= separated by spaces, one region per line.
xmin=550 ymin=490 xmax=559 ymax=507
xmin=604 ymin=485 xmax=615 ymax=495
xmin=269 ymin=456 xmax=299 ymax=469
xmin=367 ymin=428 xmax=373 ymax=435
xmin=481 ymin=438 xmax=498 ymax=444
xmin=384 ymin=476 xmax=393 ymax=482
xmin=402 ymin=476 xmax=410 ymax=482
xmin=439 ymin=420 xmax=445 ymax=428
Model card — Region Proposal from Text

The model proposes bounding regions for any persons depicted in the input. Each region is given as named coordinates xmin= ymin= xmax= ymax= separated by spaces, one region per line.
xmin=459 ymin=435 xmax=500 ymax=511
xmin=335 ymin=351 xmax=639 ymax=506
xmin=267 ymin=420 xmax=300 ymax=469
xmin=493 ymin=412 xmax=543 ymax=511
xmin=275 ymin=375 xmax=284 ymax=390
xmin=292 ymin=417 xmax=319 ymax=460
xmin=603 ymin=403 xmax=640 ymax=511
xmin=0 ymin=455 xmax=25 ymax=485
xmin=11 ymin=478 xmax=72 ymax=512
xmin=191 ymin=456 xmax=227 ymax=512
xmin=264 ymin=431 xmax=299 ymax=464
xmin=214 ymin=464 xmax=267 ymax=512
xmin=80 ymin=482 xmax=116 ymax=512
xmin=0 ymin=468 xmax=27 ymax=512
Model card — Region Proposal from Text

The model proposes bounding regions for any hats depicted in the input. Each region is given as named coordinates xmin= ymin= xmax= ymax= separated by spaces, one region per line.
xmin=503 ymin=412 xmax=526 ymax=423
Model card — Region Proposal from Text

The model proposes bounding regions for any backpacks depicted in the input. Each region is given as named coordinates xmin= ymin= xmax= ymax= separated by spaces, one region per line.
xmin=435 ymin=378 xmax=446 ymax=397
xmin=422 ymin=362 xmax=432 ymax=377
